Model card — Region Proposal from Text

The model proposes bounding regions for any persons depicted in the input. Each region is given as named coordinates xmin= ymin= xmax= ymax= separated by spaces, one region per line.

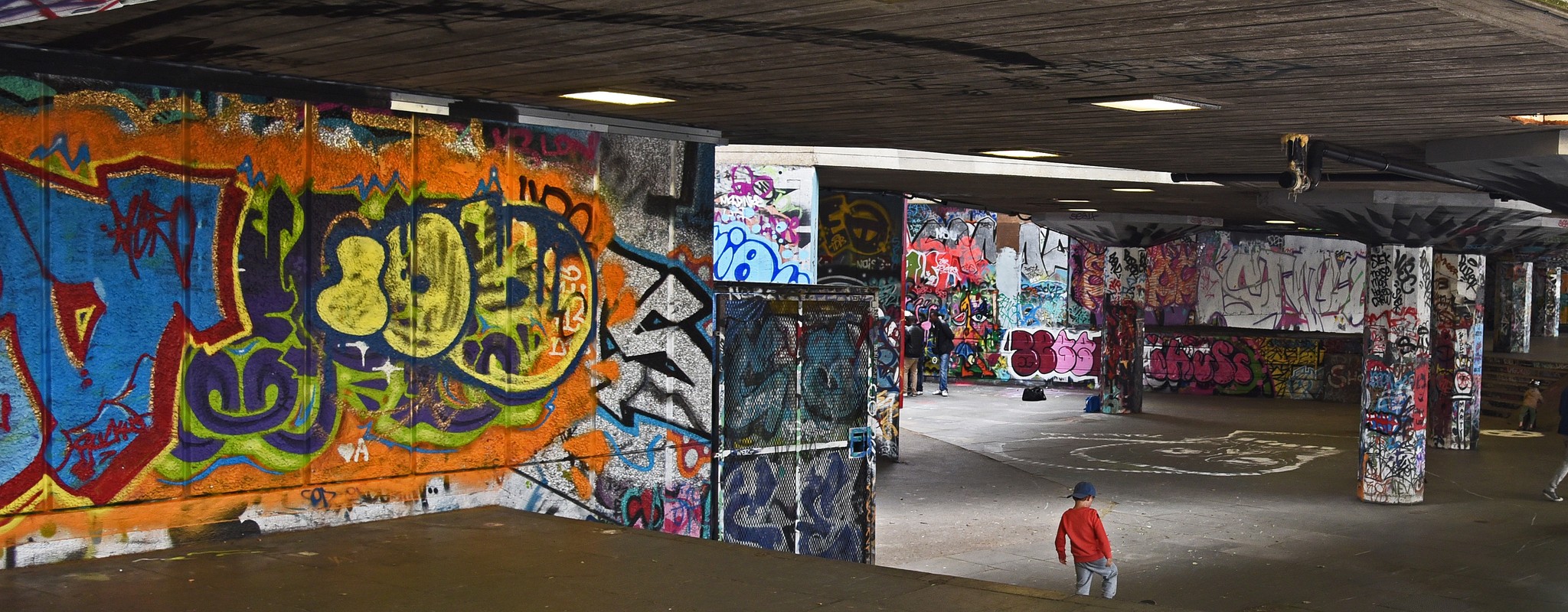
xmin=1055 ymin=482 xmax=1117 ymax=598
xmin=930 ymin=311 xmax=955 ymax=396
xmin=903 ymin=315 xmax=925 ymax=397
xmin=1518 ymin=378 xmax=1544 ymax=431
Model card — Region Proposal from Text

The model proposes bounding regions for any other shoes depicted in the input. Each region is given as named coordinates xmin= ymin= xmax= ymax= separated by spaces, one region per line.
xmin=1526 ymin=426 xmax=1533 ymax=432
xmin=917 ymin=391 xmax=923 ymax=395
xmin=910 ymin=393 xmax=916 ymax=396
xmin=903 ymin=393 xmax=907 ymax=397
xmin=1516 ymin=425 xmax=1524 ymax=430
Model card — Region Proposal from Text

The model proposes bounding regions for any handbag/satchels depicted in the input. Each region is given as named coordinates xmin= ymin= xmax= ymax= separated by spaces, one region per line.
xmin=931 ymin=344 xmax=939 ymax=354
xmin=1022 ymin=387 xmax=1046 ymax=401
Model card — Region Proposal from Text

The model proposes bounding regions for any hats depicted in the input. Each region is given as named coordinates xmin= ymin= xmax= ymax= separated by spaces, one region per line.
xmin=1067 ymin=481 xmax=1096 ymax=498
xmin=1528 ymin=379 xmax=1539 ymax=385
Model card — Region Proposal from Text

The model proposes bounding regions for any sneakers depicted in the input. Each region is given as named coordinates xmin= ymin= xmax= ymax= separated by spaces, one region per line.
xmin=1543 ymin=488 xmax=1563 ymax=501
xmin=933 ymin=390 xmax=942 ymax=395
xmin=942 ymin=390 xmax=948 ymax=396
